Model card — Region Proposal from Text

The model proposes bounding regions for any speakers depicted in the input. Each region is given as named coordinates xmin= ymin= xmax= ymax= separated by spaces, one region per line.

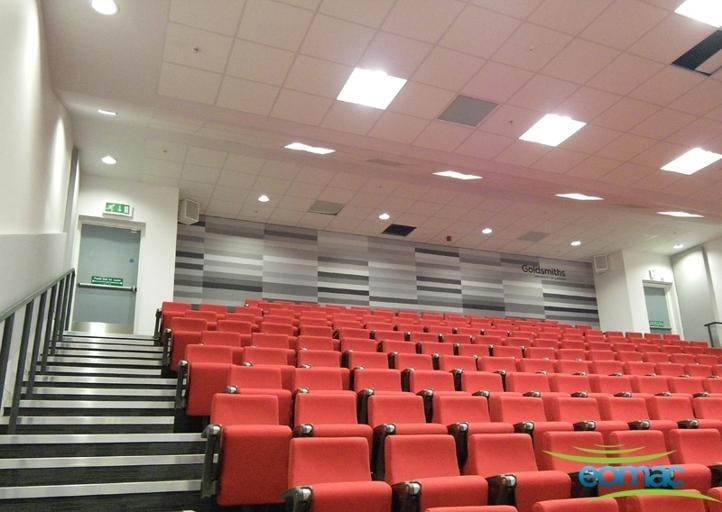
xmin=593 ymin=255 xmax=608 ymax=273
xmin=178 ymin=198 xmax=200 ymax=225
